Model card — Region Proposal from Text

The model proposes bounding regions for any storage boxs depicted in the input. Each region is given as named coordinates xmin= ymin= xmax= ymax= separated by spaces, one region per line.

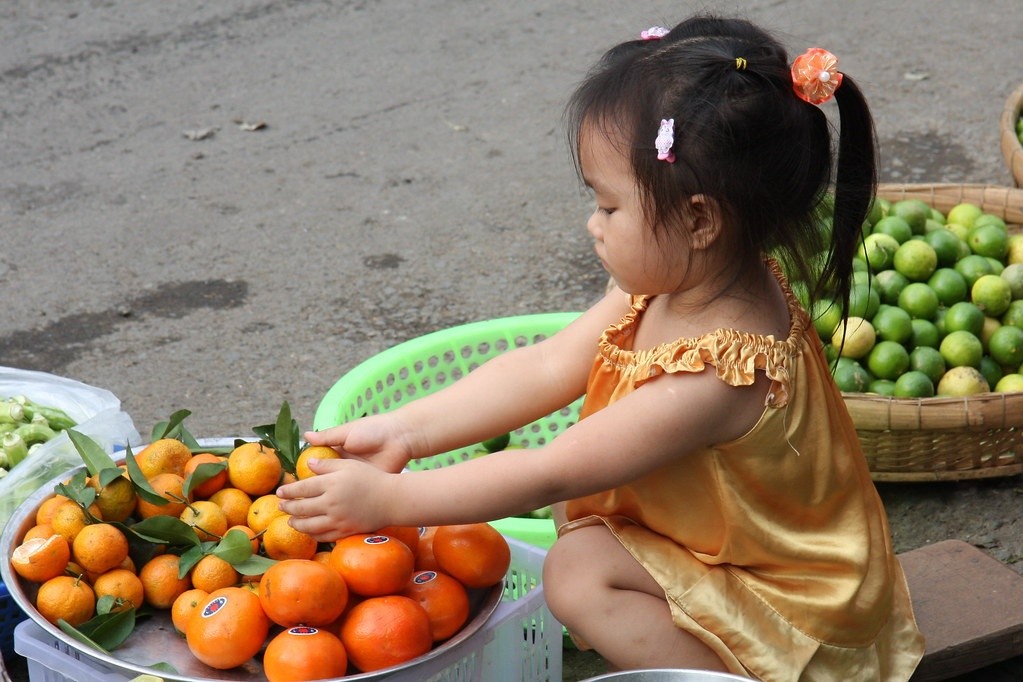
xmin=0 ymin=366 xmax=143 ymax=682
xmin=765 ymin=181 xmax=1022 ymax=483
xmin=1000 ymin=83 xmax=1023 ymax=189
xmin=14 ymin=529 xmax=564 ymax=682
xmin=313 ymin=310 xmax=596 ymax=635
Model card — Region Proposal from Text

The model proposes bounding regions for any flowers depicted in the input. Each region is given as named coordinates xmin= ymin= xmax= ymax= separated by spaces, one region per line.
xmin=791 ymin=47 xmax=843 ymax=105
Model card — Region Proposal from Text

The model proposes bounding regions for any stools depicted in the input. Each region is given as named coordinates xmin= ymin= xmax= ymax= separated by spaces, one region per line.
xmin=893 ymin=537 xmax=1022 ymax=681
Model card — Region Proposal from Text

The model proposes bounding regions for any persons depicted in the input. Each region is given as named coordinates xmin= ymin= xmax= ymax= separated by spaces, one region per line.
xmin=284 ymin=16 xmax=927 ymax=682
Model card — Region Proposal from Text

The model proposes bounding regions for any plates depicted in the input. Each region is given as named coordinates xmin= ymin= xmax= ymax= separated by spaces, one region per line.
xmin=1 ymin=436 xmax=507 ymax=682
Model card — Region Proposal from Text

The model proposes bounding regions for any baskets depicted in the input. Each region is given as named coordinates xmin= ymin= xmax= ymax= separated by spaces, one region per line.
xmin=836 ymin=180 xmax=1023 ymax=483
xmin=310 ymin=312 xmax=587 ymax=638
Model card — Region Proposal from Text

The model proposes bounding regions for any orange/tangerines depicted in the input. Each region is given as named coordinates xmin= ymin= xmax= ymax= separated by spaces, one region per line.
xmin=13 ymin=439 xmax=510 ymax=682
xmin=770 ymin=185 xmax=1023 ymax=401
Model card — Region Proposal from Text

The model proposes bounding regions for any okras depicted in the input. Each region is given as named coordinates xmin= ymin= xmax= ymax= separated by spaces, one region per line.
xmin=0 ymin=395 xmax=78 ymax=479
